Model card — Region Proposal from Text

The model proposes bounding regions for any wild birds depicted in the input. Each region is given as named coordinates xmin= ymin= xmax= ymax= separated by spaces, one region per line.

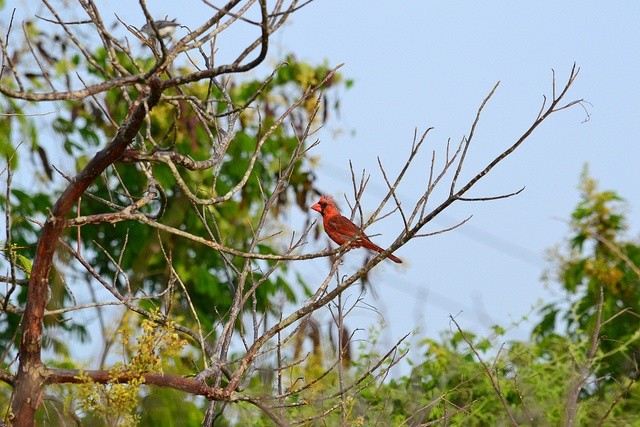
xmin=311 ymin=194 xmax=403 ymax=264
